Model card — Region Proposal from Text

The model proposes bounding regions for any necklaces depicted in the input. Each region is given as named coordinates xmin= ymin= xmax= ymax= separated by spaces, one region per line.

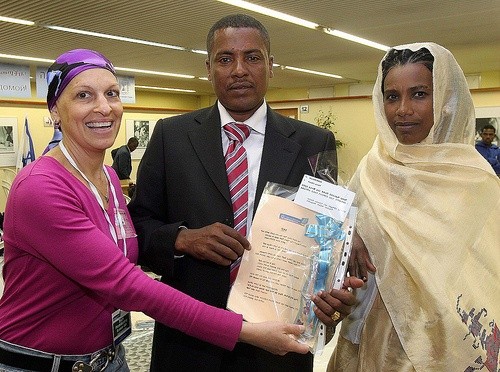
xmin=87 ymin=178 xmax=109 ymax=202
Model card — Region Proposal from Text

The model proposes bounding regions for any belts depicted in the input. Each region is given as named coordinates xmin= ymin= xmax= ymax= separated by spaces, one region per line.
xmin=0 ymin=347 xmax=114 ymax=372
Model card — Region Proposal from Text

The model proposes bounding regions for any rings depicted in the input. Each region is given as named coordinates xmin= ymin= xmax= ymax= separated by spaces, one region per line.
xmin=330 ymin=310 xmax=340 ymax=321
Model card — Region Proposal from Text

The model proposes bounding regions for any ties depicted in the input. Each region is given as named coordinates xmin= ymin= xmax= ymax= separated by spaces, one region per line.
xmin=222 ymin=122 xmax=251 ymax=286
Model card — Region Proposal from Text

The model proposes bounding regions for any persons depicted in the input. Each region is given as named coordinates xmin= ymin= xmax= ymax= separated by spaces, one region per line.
xmin=0 ymin=48 xmax=308 ymax=372
xmin=475 ymin=124 xmax=500 ymax=179
xmin=111 ymin=137 xmax=139 ymax=180
xmin=326 ymin=42 xmax=500 ymax=372
xmin=126 ymin=13 xmax=364 ymax=372
